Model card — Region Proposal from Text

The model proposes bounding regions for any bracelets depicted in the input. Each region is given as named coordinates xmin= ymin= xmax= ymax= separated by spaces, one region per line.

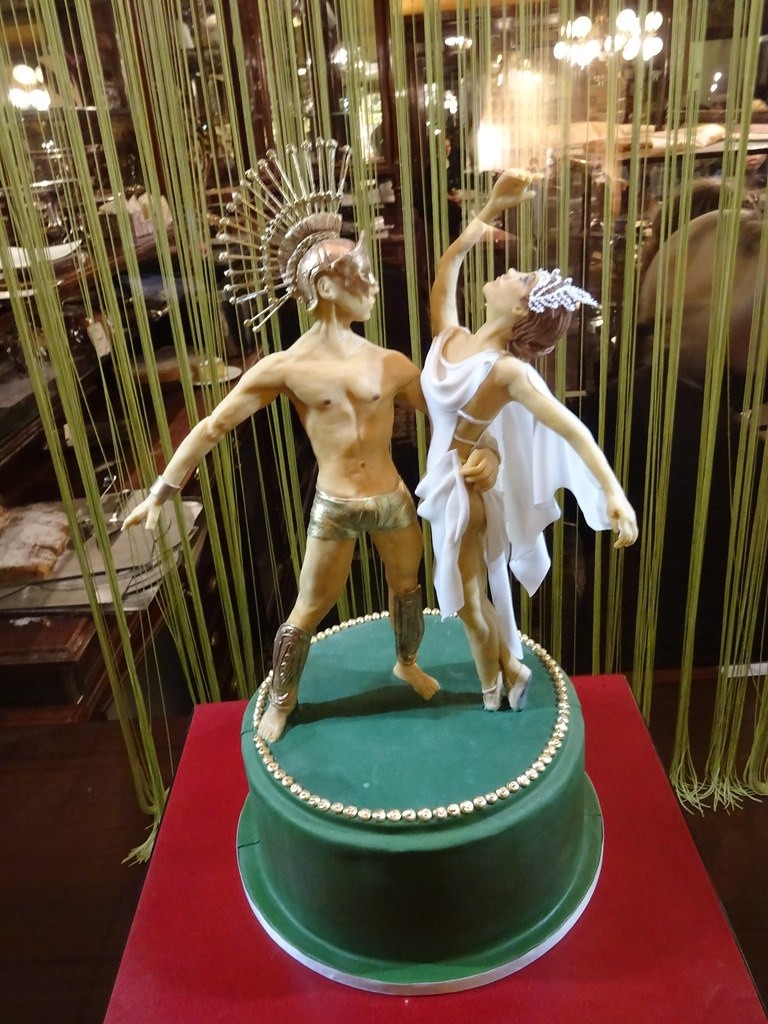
xmin=146 ymin=477 xmax=180 ymax=501
xmin=478 ymin=436 xmax=501 ymax=462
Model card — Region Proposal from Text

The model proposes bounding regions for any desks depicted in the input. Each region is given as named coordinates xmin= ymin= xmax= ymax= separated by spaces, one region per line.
xmin=1 ymin=225 xmax=317 ymax=725
xmin=102 ymin=673 xmax=767 ymax=1024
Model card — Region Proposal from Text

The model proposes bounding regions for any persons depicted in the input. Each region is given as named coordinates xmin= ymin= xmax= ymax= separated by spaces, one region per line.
xmin=415 ymin=166 xmax=638 ymax=715
xmin=421 ymin=137 xmax=463 ymax=238
xmin=118 ymin=135 xmax=504 ymax=743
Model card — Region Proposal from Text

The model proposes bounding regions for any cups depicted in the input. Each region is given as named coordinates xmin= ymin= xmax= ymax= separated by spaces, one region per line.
xmin=198 ymin=357 xmax=224 ymax=380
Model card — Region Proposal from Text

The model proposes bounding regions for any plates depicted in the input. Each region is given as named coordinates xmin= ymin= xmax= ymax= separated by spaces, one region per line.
xmin=722 ymin=131 xmax=767 ymax=142
xmin=180 ymin=366 xmax=241 ymax=385
xmin=0 ymin=237 xmax=82 ymax=269
xmin=0 ymin=278 xmax=64 ymax=298
